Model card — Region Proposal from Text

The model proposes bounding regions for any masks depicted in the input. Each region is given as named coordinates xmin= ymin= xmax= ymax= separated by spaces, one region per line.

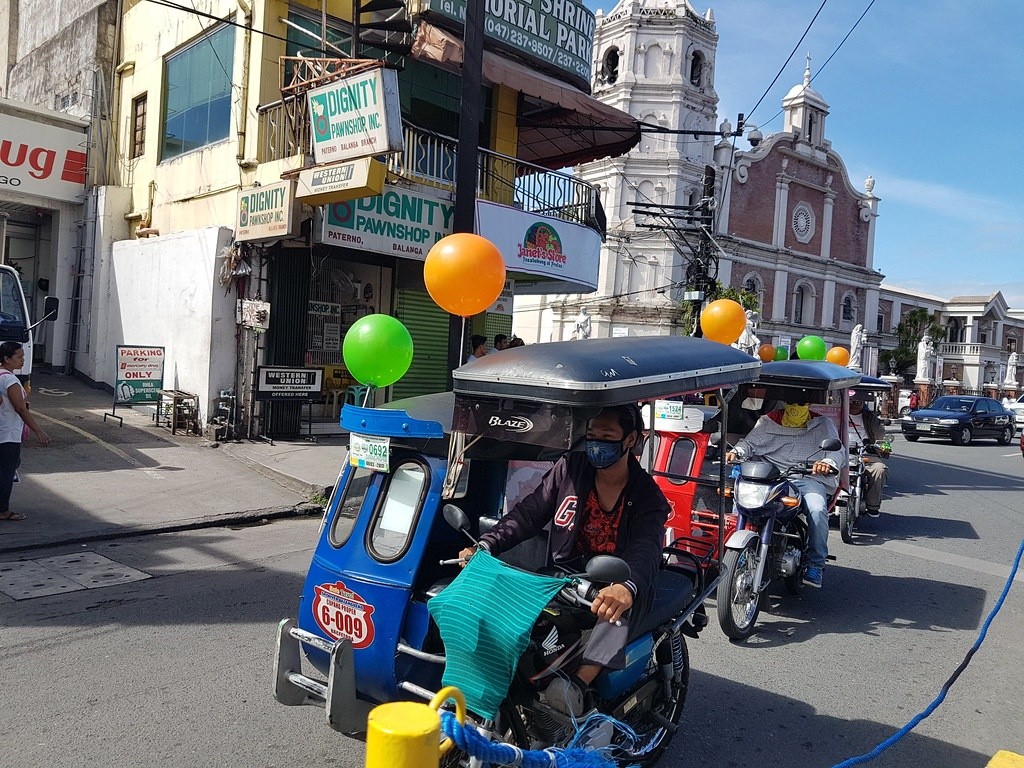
xmin=585 ymin=438 xmax=628 ymax=469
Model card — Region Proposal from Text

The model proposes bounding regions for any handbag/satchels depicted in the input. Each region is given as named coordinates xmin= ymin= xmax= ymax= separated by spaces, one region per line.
xmin=862 ymin=439 xmax=876 ymax=454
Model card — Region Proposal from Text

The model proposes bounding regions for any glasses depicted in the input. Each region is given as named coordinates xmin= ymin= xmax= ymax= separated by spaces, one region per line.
xmin=850 ymin=399 xmax=862 ymax=406
xmin=785 ymin=401 xmax=805 ymax=406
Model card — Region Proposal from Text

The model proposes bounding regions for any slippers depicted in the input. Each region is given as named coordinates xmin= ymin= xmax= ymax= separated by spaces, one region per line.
xmin=0 ymin=512 xmax=27 ymax=521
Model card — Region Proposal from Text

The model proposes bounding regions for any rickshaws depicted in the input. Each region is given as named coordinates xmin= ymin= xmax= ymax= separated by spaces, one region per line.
xmin=271 ymin=333 xmax=761 ymax=768
xmin=828 ymin=373 xmax=894 ymax=543
xmin=637 ymin=358 xmax=862 ymax=640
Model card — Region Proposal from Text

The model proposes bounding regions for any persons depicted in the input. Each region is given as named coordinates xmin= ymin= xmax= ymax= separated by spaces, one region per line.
xmin=847 ymin=394 xmax=890 ymax=518
xmin=721 ymin=389 xmax=848 ymax=588
xmin=572 ymin=307 xmax=592 ymax=340
xmin=738 ymin=310 xmax=762 ymax=361
xmin=919 ymin=336 xmax=934 ymax=378
xmin=1004 ymin=352 xmax=1019 ymax=385
xmin=465 ymin=330 xmax=527 ymax=369
xmin=907 ymin=386 xmax=920 ymax=412
xmin=460 ymin=397 xmax=675 ymax=711
xmin=1 ymin=342 xmax=53 ymax=520
xmin=849 ymin=324 xmax=863 ymax=366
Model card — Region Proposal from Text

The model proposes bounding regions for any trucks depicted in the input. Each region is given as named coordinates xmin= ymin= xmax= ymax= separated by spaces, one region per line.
xmin=0 ymin=262 xmax=60 ymax=398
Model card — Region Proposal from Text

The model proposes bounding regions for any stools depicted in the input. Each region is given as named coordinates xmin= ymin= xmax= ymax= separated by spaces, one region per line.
xmin=323 ymin=390 xmax=344 ymax=419
xmin=157 ymin=389 xmax=198 ymax=436
xmin=344 ymin=385 xmax=371 ymax=408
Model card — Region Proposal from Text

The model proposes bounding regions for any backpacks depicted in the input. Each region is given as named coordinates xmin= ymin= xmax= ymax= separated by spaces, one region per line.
xmin=910 ymin=394 xmax=918 ymax=409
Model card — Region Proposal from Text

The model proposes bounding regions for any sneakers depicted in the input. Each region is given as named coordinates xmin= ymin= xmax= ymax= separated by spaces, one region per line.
xmin=802 ymin=567 xmax=823 ymax=588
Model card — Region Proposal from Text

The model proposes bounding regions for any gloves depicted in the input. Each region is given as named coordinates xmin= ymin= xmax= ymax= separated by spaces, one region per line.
xmin=880 ymin=443 xmax=891 ymax=454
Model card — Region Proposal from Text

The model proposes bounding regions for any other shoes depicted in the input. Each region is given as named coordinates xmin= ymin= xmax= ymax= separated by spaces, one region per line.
xmin=546 ymin=678 xmax=589 ymax=717
xmin=866 ymin=508 xmax=880 ymax=517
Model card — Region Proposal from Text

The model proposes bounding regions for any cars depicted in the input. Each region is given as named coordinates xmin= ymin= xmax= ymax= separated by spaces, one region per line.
xmin=900 ymin=395 xmax=1018 ymax=447
xmin=865 ymin=389 xmax=913 ymax=418
xmin=1009 ymin=394 xmax=1024 ymax=429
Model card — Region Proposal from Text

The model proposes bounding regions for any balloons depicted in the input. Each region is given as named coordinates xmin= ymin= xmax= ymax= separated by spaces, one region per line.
xmin=343 ymin=313 xmax=414 ymax=389
xmin=797 ymin=335 xmax=827 ymax=361
xmin=758 ymin=343 xmax=776 ymax=363
xmin=422 ymin=232 xmax=507 ymax=316
xmin=700 ymin=299 xmax=747 ymax=346
xmin=772 ymin=346 xmax=788 ymax=362
xmin=826 ymin=347 xmax=850 ymax=367
